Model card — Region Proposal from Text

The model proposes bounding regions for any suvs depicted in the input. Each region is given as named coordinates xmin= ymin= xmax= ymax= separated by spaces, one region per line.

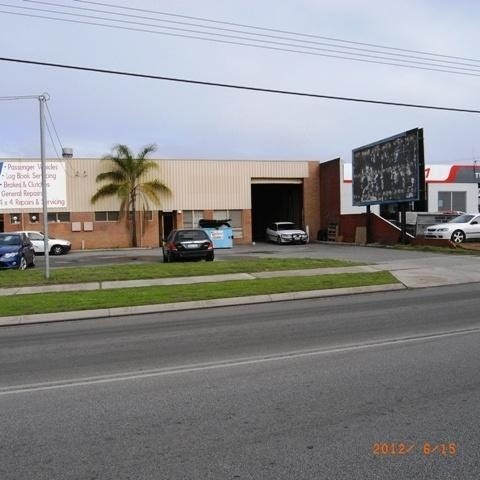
xmin=0 ymin=231 xmax=36 ymax=273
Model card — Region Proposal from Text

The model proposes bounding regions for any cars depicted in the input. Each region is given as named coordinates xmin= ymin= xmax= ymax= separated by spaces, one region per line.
xmin=266 ymin=221 xmax=308 ymax=245
xmin=11 ymin=230 xmax=73 ymax=257
xmin=423 ymin=212 xmax=480 ymax=245
xmin=160 ymin=227 xmax=215 ymax=263
